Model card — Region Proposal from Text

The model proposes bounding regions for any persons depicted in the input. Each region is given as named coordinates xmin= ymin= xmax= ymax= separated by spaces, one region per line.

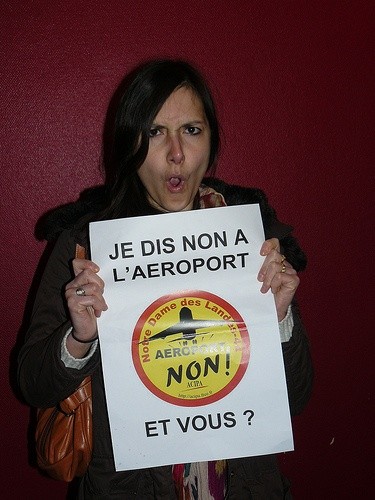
xmin=8 ymin=57 xmax=313 ymax=500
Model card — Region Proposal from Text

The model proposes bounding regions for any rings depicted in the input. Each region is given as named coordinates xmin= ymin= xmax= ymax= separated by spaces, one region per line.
xmin=280 ymin=254 xmax=286 ymax=263
xmin=75 ymin=287 xmax=86 ymax=296
xmin=282 ymin=264 xmax=286 ymax=273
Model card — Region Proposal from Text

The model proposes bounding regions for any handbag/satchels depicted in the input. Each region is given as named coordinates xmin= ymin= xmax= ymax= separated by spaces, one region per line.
xmin=33 ymin=375 xmax=93 ymax=484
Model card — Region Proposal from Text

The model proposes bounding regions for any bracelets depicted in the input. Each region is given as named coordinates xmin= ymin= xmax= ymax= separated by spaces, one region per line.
xmin=72 ymin=329 xmax=98 ymax=343
